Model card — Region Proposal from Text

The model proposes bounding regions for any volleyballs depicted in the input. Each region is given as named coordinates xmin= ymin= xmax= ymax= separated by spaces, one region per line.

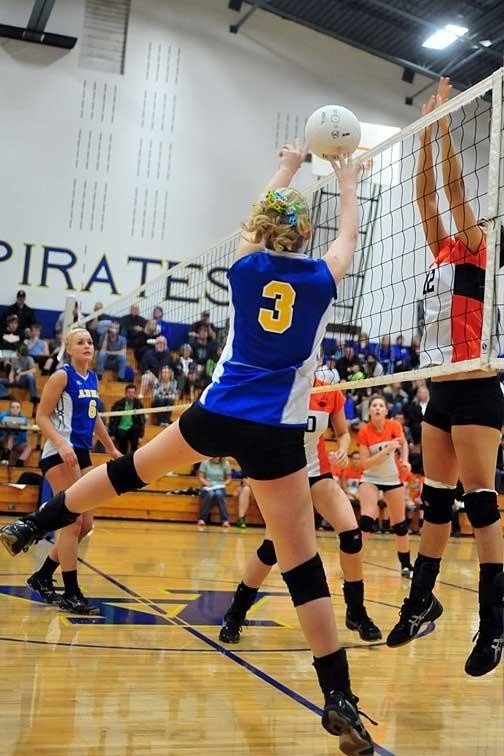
xmin=304 ymin=106 xmax=360 ymax=158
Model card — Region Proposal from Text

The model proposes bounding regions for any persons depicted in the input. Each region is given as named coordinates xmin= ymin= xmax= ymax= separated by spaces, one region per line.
xmin=27 ymin=328 xmax=128 ymax=613
xmin=357 ymin=394 xmax=413 ymax=579
xmin=1 ymin=139 xmax=383 ymax=754
xmin=385 ymin=76 xmax=504 ymax=676
xmin=217 ymin=349 xmax=381 ymax=644
xmin=0 ymin=290 xmax=503 ymax=538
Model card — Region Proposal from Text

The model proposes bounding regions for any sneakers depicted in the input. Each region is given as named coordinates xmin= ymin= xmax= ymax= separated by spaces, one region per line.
xmin=387 ymin=592 xmax=443 ymax=646
xmin=464 ymin=620 xmax=504 ymax=676
xmin=58 ymin=594 xmax=101 ymax=616
xmin=27 ymin=572 xmax=61 ymax=603
xmin=0 ymin=518 xmax=42 ymax=555
xmin=321 ymin=690 xmax=373 ymax=756
xmin=219 ymin=608 xmax=242 ymax=643
xmin=346 ymin=609 xmax=381 ymax=639
xmin=401 ymin=564 xmax=413 ymax=579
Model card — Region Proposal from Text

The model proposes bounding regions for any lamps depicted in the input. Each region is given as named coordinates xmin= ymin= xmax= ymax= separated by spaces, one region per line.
xmin=420 ymin=17 xmax=474 ymax=51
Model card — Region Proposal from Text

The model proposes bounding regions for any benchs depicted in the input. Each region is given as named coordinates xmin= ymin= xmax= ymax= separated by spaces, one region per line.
xmin=0 ymin=333 xmax=504 ymax=538
